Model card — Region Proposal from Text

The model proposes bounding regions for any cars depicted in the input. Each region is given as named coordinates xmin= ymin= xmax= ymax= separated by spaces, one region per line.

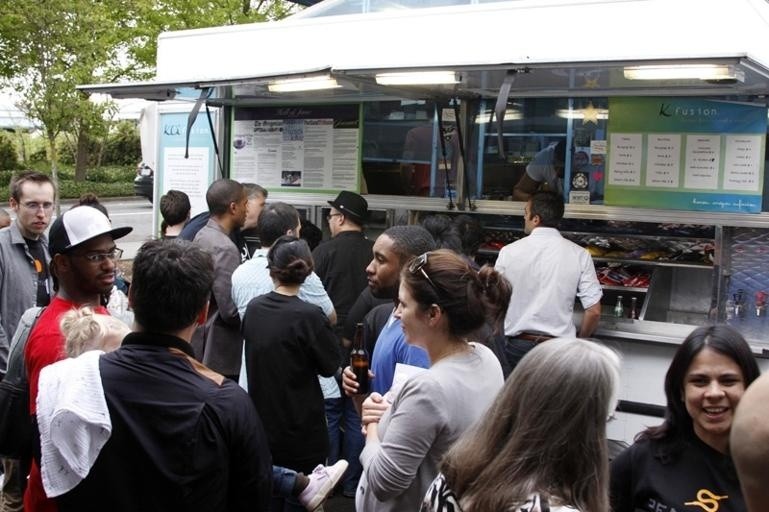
xmin=134 ymin=160 xmax=154 ymax=204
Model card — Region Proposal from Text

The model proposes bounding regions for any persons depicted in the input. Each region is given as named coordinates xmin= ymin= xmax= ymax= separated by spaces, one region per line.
xmin=729 ymin=371 xmax=768 ymax=511
xmin=231 ymin=202 xmax=342 ymax=501
xmin=160 ymin=189 xmax=191 ymax=238
xmin=24 ymin=203 xmax=133 ymax=511
xmin=178 ymin=182 xmax=268 ymax=249
xmin=312 ymin=191 xmax=376 ymax=328
xmin=191 ymin=175 xmax=244 ymax=379
xmin=158 ymin=220 xmax=171 ymax=237
xmin=493 ymin=190 xmax=603 ymax=372
xmin=419 ymin=337 xmax=622 ymax=512
xmin=355 ymin=248 xmax=513 ymax=511
xmin=300 ymin=218 xmax=323 ymax=253
xmin=239 ymin=236 xmax=347 ymax=511
xmin=1 ymin=208 xmax=11 ymax=227
xmin=285 ymin=171 xmax=300 ymax=185
xmin=67 ymin=193 xmax=130 ymax=298
xmin=609 ymin=324 xmax=760 ymax=511
xmin=423 ymin=213 xmax=484 ymax=264
xmin=401 ymin=98 xmax=447 ymax=196
xmin=30 ymin=237 xmax=273 ymax=511
xmin=2 ymin=258 xmax=51 ymax=512
xmin=341 ymin=226 xmax=442 ymax=418
xmin=60 ymin=305 xmax=350 ymax=512
xmin=513 ymin=138 xmax=576 ymax=202
xmin=0 ymin=171 xmax=56 ymax=510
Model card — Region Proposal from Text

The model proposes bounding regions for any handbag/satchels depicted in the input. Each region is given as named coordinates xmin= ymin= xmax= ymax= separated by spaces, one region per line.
xmin=0 ymin=306 xmax=43 ymax=459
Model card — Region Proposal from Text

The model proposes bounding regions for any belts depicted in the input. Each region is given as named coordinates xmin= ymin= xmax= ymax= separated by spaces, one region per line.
xmin=509 ymin=332 xmax=554 ymax=343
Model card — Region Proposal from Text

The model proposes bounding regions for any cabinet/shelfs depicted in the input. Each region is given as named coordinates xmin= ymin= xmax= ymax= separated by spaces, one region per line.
xmin=473 ymin=215 xmax=715 ymax=322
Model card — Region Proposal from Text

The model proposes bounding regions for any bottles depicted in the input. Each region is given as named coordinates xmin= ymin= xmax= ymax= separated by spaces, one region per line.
xmin=613 ymin=296 xmax=622 ymax=316
xmin=630 ymin=297 xmax=638 ymax=320
xmin=348 ymin=321 xmax=369 ymax=393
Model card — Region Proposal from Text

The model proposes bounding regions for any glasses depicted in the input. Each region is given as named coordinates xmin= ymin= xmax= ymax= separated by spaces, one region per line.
xmin=408 ymin=252 xmax=448 ymax=315
xmin=17 ymin=201 xmax=55 ymax=209
xmin=326 ymin=213 xmax=341 ymax=220
xmin=63 ymin=247 xmax=124 ymax=264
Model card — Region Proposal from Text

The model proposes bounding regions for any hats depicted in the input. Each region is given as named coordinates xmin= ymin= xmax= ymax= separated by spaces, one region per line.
xmin=327 ymin=189 xmax=371 ymax=224
xmin=47 ymin=203 xmax=133 ymax=253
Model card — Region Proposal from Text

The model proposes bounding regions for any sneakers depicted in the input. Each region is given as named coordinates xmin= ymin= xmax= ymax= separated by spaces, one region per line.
xmin=298 ymin=459 xmax=349 ymax=512
